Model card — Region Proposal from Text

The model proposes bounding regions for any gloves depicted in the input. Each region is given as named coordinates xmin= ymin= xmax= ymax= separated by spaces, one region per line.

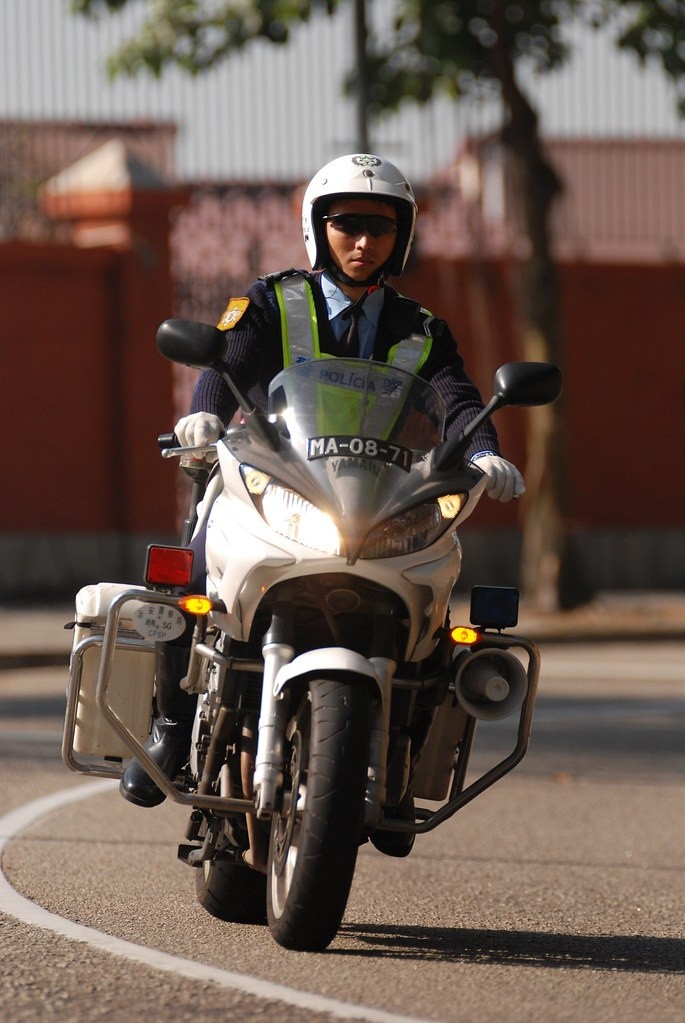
xmin=469 ymin=453 xmax=526 ymax=504
xmin=174 ymin=411 xmax=226 ymax=459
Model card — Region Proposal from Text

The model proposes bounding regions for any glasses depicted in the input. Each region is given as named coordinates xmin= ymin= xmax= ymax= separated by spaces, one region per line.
xmin=321 ymin=211 xmax=403 ymax=236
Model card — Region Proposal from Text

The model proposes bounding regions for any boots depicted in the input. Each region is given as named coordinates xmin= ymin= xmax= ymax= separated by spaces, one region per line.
xmin=120 ymin=646 xmax=193 ymax=807
xmin=371 ymin=733 xmax=417 ymax=858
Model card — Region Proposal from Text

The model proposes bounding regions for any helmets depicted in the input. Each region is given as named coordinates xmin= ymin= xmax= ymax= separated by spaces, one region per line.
xmin=301 ymin=153 xmax=418 ymax=276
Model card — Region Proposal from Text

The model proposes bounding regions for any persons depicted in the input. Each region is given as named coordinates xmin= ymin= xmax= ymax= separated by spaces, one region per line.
xmin=121 ymin=153 xmax=527 ymax=857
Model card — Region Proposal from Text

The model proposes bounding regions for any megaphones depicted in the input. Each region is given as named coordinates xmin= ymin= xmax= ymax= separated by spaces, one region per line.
xmin=452 ymin=646 xmax=529 ymax=721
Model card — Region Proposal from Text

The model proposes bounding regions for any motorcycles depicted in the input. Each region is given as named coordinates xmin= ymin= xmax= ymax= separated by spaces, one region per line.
xmin=59 ymin=320 xmax=565 ymax=953
